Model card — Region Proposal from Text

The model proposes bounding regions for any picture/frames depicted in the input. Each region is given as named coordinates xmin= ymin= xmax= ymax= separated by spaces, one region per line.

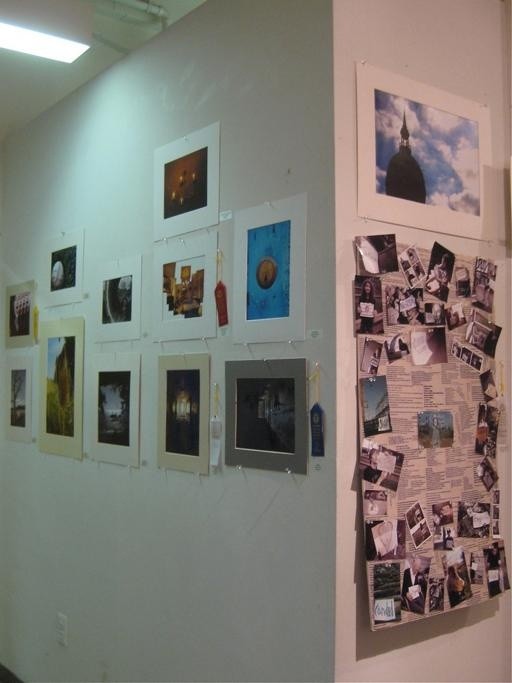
xmin=156 ymin=354 xmax=212 ymax=476
xmin=1 ymin=278 xmax=38 ymax=348
xmin=224 ymin=358 xmax=309 ymax=475
xmin=152 ymin=121 xmax=220 ymax=241
xmin=38 ymin=229 xmax=85 ymax=307
xmin=89 ymin=255 xmax=142 ymax=344
xmin=1 ymin=355 xmax=35 ymax=439
xmin=357 ymin=60 xmax=494 ymax=243
xmin=37 ymin=314 xmax=86 ymax=459
xmin=228 ymin=192 xmax=307 ymax=344
xmin=149 ymin=234 xmax=217 ymax=343
